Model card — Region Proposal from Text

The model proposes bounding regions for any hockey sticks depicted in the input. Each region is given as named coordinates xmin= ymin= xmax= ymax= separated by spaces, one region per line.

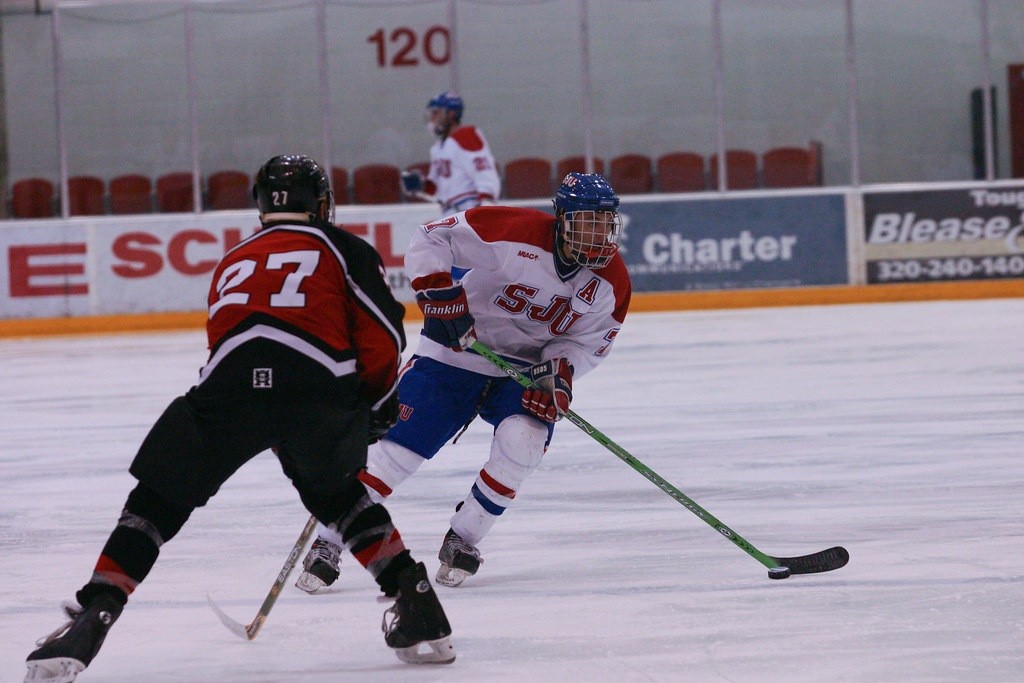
xmin=465 ymin=334 xmax=851 ymax=576
xmin=204 ymin=515 xmax=318 ymax=643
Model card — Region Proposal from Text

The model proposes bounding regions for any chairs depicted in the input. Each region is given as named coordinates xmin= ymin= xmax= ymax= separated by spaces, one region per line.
xmin=156 ymin=173 xmax=196 ymax=213
xmin=711 ymin=151 xmax=758 ymax=193
xmin=505 ymin=158 xmax=553 ymax=201
xmin=11 ymin=176 xmax=57 ymax=217
xmin=109 ymin=174 xmax=152 ymax=214
xmin=610 ymin=156 xmax=652 ymax=197
xmin=208 ymin=171 xmax=250 ymax=210
xmin=559 ymin=157 xmax=605 ymax=203
xmin=66 ymin=174 xmax=105 ymax=215
xmin=661 ymin=153 xmax=705 ymax=194
xmin=764 ymin=141 xmax=826 ymax=189
xmin=352 ymin=163 xmax=401 ymax=205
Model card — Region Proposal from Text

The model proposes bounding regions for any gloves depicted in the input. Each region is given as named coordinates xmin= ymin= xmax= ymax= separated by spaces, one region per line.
xmin=521 ymin=358 xmax=574 ymax=424
xmin=414 ymin=281 xmax=478 ymax=353
xmin=401 ymin=174 xmax=423 ymax=192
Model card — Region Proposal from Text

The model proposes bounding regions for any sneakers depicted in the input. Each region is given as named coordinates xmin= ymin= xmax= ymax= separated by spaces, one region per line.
xmin=433 ymin=530 xmax=485 ymax=588
xmin=380 ymin=563 xmax=459 ymax=665
xmin=22 ymin=582 xmax=128 ymax=683
xmin=295 ymin=536 xmax=343 ymax=592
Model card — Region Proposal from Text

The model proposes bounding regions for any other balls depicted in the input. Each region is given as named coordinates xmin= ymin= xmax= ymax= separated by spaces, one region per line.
xmin=767 ymin=567 xmax=791 ymax=579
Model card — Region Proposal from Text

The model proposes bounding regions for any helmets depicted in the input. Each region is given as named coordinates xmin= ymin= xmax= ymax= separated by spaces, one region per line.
xmin=252 ymin=154 xmax=336 ymax=222
xmin=551 ymin=172 xmax=622 ymax=271
xmin=425 ymin=93 xmax=464 ymax=136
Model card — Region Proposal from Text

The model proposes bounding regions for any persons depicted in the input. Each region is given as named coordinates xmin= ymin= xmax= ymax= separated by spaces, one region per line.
xmin=401 ymin=91 xmax=501 ymax=213
xmin=26 ymin=154 xmax=453 ymax=669
xmin=303 ymin=173 xmax=633 ymax=587
